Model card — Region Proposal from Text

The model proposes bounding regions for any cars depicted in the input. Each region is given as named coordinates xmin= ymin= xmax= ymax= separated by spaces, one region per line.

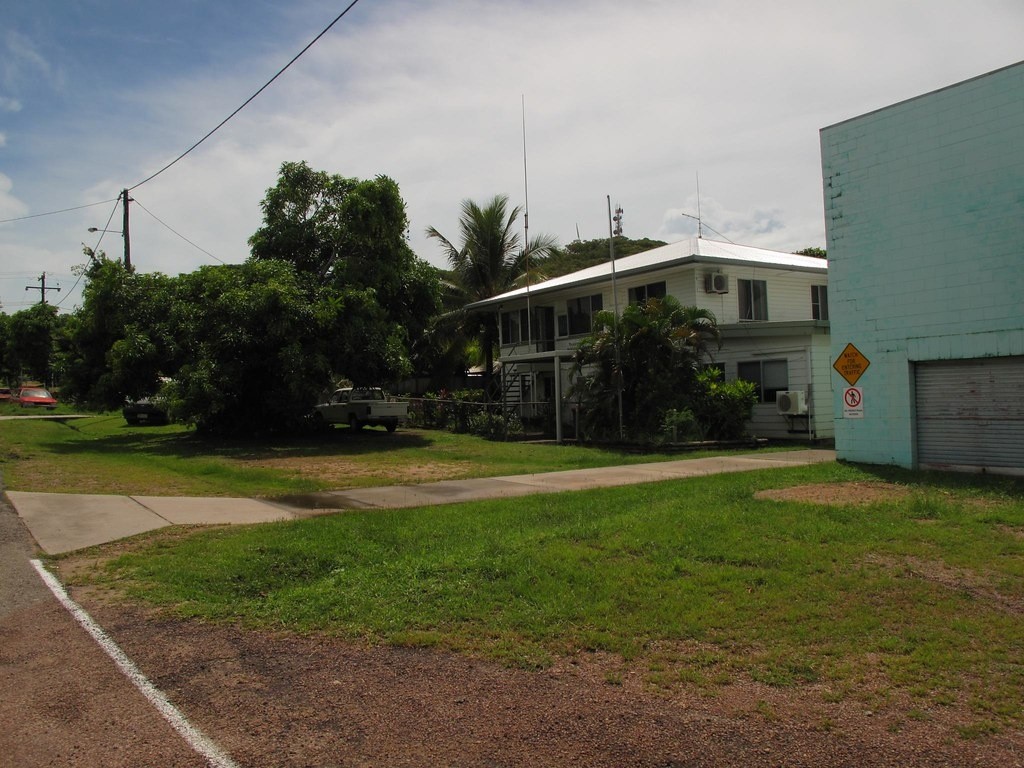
xmin=127 ymin=397 xmax=175 ymax=426
xmin=0 ymin=388 xmax=12 ymax=401
xmin=9 ymin=387 xmax=57 ymax=410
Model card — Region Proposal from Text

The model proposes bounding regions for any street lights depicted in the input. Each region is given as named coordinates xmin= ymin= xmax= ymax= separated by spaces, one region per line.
xmin=88 ymin=227 xmax=136 ymax=276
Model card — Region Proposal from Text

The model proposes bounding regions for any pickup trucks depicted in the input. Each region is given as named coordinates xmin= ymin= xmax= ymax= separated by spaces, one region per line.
xmin=313 ymin=386 xmax=409 ymax=432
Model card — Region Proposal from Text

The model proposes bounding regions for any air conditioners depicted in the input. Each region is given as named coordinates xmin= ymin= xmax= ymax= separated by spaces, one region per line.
xmin=705 ymin=271 xmax=728 ymax=294
xmin=777 ymin=390 xmax=808 ymax=413
